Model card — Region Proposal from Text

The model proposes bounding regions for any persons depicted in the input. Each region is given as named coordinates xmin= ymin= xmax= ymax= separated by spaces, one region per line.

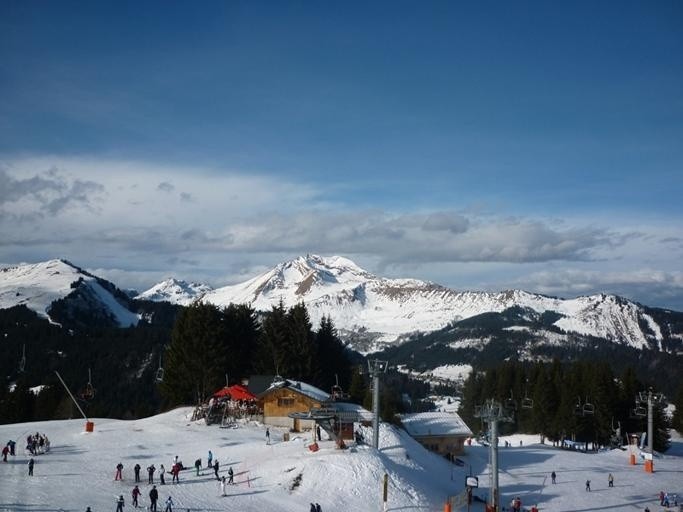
xmin=131 ymin=484 xmax=141 ymax=508
xmin=316 ymin=425 xmax=321 ymax=440
xmin=531 ymin=503 xmax=538 ymax=512
xmin=26 ymin=458 xmax=33 ymax=476
xmin=171 ymin=455 xmax=185 ymax=470
xmin=146 ymin=463 xmax=156 ymax=484
xmin=206 ymin=449 xmax=212 ymax=466
xmin=643 ymin=506 xmax=650 ymax=512
xmin=550 ymin=470 xmax=555 ymax=484
xmin=585 ymin=479 xmax=591 ymax=491
xmin=309 ymin=502 xmax=315 ymax=511
xmin=513 ymin=496 xmax=521 ymax=512
xmin=226 ymin=465 xmax=233 ymax=483
xmin=6 ymin=439 xmax=15 ymax=456
xmin=658 ymin=490 xmax=664 ymax=505
xmin=212 ymin=459 xmax=220 ymax=480
xmin=115 ymin=495 xmax=124 ymax=512
xmin=84 ymin=506 xmax=92 ymax=512
xmin=314 ymin=502 xmax=322 ymax=511
xmin=194 ymin=456 xmax=201 ymax=476
xmin=157 ymin=463 xmax=166 ymax=485
xmin=163 ymin=495 xmax=174 ymax=512
xmin=170 ymin=463 xmax=180 ymax=482
xmin=220 ymin=475 xmax=226 ymax=496
xmin=207 ymin=396 xmax=217 ymax=416
xmin=148 ymin=485 xmax=158 ymax=512
xmin=1 ymin=446 xmax=9 ymax=462
xmin=607 ymin=472 xmax=614 ymax=487
xmin=662 ymin=492 xmax=670 ymax=507
xmin=265 ymin=427 xmax=271 ymax=445
xmin=25 ymin=431 xmax=49 ymax=456
xmin=133 ymin=463 xmax=141 ymax=483
xmin=114 ymin=461 xmax=123 ymax=481
